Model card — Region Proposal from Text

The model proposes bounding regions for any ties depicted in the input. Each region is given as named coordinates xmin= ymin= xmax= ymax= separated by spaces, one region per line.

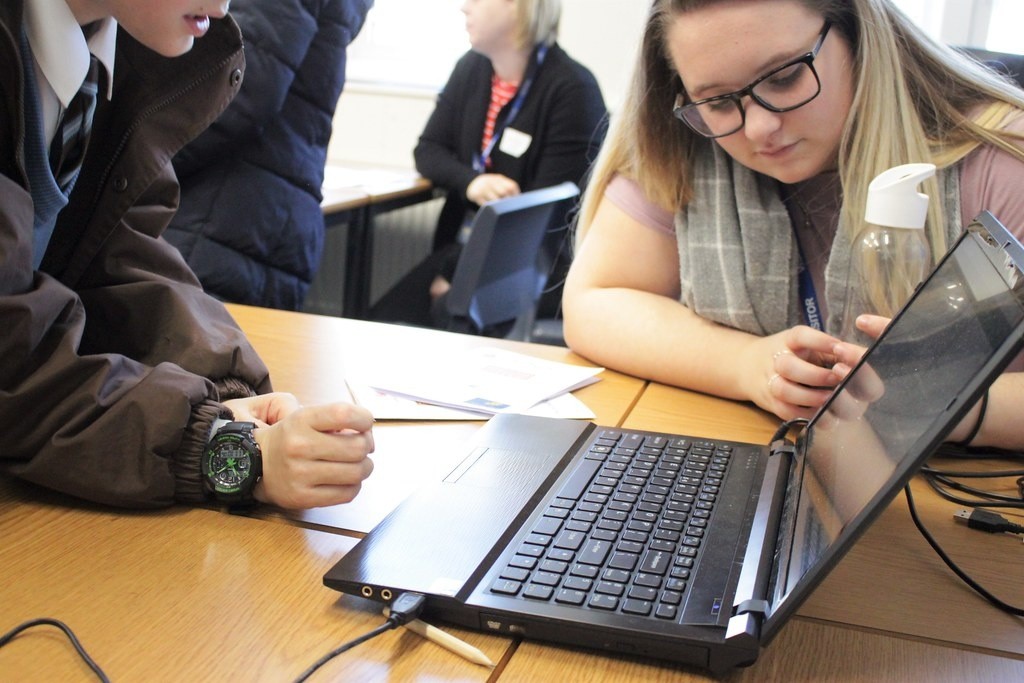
xmin=55 ymin=55 xmax=102 ymax=195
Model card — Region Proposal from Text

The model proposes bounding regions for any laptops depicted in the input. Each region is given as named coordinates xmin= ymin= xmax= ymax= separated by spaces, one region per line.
xmin=317 ymin=211 xmax=1024 ymax=679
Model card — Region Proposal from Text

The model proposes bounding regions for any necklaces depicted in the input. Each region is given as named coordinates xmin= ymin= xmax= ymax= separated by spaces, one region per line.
xmin=791 ymin=185 xmax=843 ymax=227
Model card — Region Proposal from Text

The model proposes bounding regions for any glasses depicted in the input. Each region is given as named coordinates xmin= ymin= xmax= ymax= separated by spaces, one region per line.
xmin=669 ymin=9 xmax=837 ymax=141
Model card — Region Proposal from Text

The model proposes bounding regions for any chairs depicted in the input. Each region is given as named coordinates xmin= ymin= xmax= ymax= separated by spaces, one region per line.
xmin=433 ymin=182 xmax=579 ymax=342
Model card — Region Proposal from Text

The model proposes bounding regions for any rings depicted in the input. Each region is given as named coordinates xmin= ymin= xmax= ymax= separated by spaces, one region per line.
xmin=768 ymin=374 xmax=781 ymax=393
xmin=772 ymin=350 xmax=791 ymax=369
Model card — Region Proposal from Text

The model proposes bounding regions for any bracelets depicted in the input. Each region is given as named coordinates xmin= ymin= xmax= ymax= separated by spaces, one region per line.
xmin=956 ymin=388 xmax=989 ymax=447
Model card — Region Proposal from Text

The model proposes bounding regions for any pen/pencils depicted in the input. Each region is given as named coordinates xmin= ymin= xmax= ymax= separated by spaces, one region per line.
xmin=383 ymin=606 xmax=496 ymax=667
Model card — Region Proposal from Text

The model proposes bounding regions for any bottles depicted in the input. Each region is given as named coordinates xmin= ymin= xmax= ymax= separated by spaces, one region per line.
xmin=838 ymin=162 xmax=938 ymax=367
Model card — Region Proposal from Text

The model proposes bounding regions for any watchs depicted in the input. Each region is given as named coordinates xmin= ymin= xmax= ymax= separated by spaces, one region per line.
xmin=200 ymin=421 xmax=263 ymax=512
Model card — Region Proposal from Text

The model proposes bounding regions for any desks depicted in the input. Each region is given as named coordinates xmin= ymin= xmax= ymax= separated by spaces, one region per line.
xmin=0 ymin=160 xmax=1024 ymax=683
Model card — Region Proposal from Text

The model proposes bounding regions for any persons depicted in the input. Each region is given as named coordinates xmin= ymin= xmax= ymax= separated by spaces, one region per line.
xmin=362 ymin=0 xmax=610 ymax=340
xmin=161 ymin=0 xmax=375 ymax=313
xmin=0 ymin=0 xmax=375 ymax=511
xmin=561 ymin=0 xmax=1024 ymax=450
